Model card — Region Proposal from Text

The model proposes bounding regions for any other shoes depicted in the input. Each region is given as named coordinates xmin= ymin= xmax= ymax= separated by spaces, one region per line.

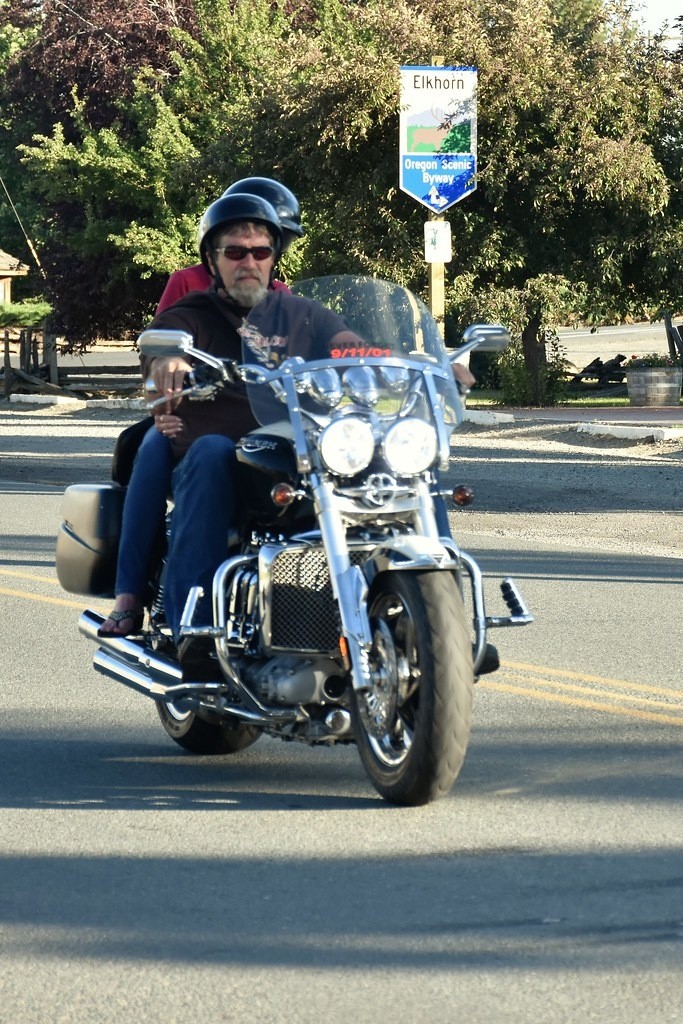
xmin=472 ymin=643 xmax=499 ymax=673
xmin=179 ymin=632 xmax=206 ymax=678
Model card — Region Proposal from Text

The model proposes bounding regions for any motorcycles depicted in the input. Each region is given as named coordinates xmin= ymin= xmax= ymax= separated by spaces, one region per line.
xmin=53 ymin=275 xmax=534 ymax=807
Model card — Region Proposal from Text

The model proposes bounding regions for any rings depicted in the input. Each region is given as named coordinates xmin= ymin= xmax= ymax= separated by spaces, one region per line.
xmin=162 ymin=430 xmax=166 ymax=436
xmin=160 ymin=416 xmax=163 ymax=423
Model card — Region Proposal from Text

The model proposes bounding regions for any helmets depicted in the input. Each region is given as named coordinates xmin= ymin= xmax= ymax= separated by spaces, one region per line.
xmin=221 ymin=177 xmax=303 ymax=238
xmin=196 ymin=193 xmax=282 ymax=276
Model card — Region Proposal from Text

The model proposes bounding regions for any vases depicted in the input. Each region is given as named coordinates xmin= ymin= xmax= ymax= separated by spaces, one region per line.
xmin=625 ymin=368 xmax=683 ymax=406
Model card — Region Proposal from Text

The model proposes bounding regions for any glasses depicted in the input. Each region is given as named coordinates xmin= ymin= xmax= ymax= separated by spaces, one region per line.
xmin=215 ymin=244 xmax=274 ymax=261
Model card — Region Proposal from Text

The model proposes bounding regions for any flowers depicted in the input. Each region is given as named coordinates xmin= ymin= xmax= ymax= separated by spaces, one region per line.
xmin=623 ymin=353 xmax=683 ymax=368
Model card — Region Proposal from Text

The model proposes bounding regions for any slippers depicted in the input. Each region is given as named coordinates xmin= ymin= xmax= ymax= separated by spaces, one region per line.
xmin=98 ymin=604 xmax=146 ymax=637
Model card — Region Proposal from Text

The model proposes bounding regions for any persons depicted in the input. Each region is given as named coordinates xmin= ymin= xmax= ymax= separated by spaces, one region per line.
xmin=98 ymin=176 xmax=501 ymax=674
